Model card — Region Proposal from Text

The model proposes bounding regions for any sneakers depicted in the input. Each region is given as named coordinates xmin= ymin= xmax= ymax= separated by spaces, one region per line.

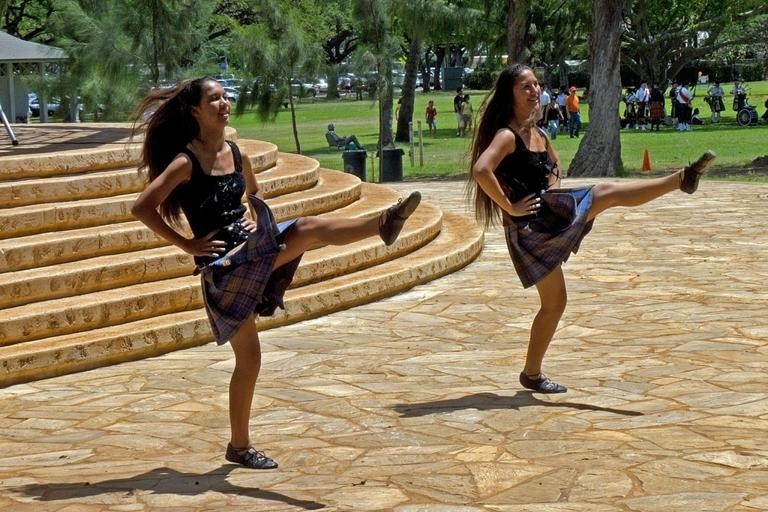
xmin=225 ymin=440 xmax=280 ymax=468
xmin=379 ymin=192 xmax=422 ymax=246
xmin=519 ymin=372 xmax=568 ymax=393
xmin=679 ymin=150 xmax=715 ymax=194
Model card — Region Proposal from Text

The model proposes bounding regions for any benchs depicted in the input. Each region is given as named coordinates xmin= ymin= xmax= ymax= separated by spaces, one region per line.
xmin=325 ymin=133 xmax=346 ymax=151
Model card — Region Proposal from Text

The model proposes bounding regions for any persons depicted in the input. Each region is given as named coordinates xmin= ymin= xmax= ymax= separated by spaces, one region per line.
xmin=453 ymin=87 xmax=466 ymax=136
xmin=354 ymin=77 xmax=363 ymax=100
xmin=394 ymin=98 xmax=402 ymax=120
xmin=129 ymin=74 xmax=423 ymax=470
xmin=327 ymin=123 xmax=364 ymax=150
xmin=461 ymin=61 xmax=716 ymax=393
xmin=534 ymin=83 xmax=582 ymax=140
xmin=620 ymin=79 xmax=746 ymax=132
xmin=460 ymin=94 xmax=474 ymax=132
xmin=425 ymin=100 xmax=438 ymax=136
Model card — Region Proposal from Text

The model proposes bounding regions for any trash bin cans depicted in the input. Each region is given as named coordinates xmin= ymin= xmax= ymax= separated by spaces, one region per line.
xmin=376 ymin=149 xmax=404 ymax=182
xmin=342 ymin=150 xmax=367 ymax=182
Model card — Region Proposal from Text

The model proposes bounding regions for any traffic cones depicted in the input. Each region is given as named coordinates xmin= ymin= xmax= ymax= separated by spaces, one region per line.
xmin=639 ymin=150 xmax=652 ymax=175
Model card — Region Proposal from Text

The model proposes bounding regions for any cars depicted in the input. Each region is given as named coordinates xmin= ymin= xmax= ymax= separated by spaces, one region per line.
xmin=27 ymin=91 xmax=106 ymax=118
xmin=150 ymin=79 xmax=178 ymax=92
xmin=213 ymin=67 xmax=443 ymax=103
